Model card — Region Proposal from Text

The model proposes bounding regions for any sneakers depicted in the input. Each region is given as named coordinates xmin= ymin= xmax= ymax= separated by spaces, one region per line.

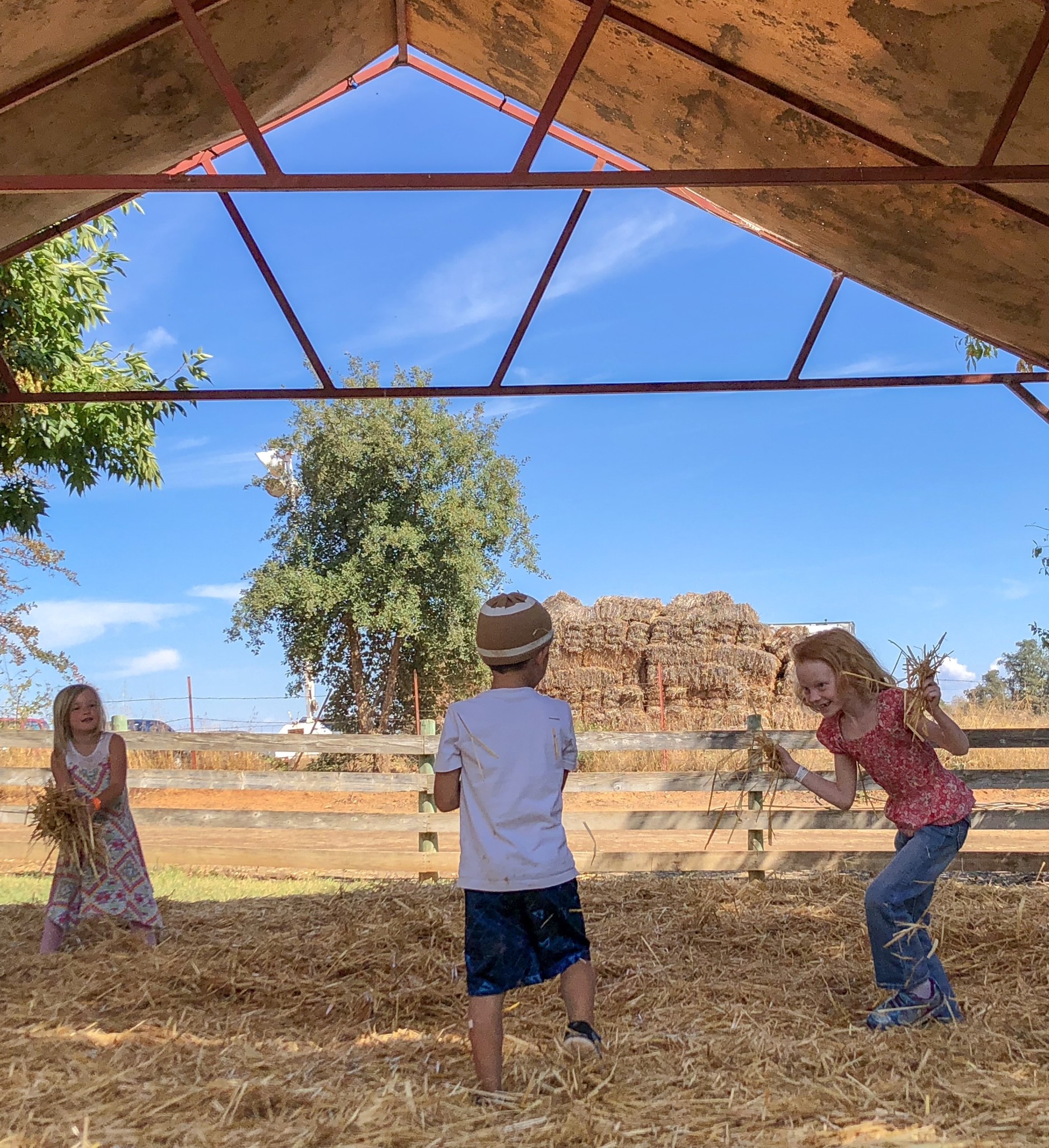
xmin=867 ymin=978 xmax=960 ymax=1030
xmin=473 ymin=1090 xmax=488 ymax=1108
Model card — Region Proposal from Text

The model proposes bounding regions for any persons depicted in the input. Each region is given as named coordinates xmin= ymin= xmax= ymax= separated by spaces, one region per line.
xmin=766 ymin=627 xmax=975 ymax=1029
xmin=432 ymin=592 xmax=606 ymax=1105
xmin=39 ymin=683 xmax=160 ymax=958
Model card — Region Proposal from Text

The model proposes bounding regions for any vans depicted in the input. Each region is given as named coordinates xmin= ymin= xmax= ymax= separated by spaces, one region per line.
xmin=127 ymin=719 xmax=177 ymax=732
xmin=0 ymin=717 xmax=50 ymax=730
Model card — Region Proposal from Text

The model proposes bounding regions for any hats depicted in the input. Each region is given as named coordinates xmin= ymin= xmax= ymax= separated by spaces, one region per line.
xmin=476 ymin=592 xmax=554 ymax=666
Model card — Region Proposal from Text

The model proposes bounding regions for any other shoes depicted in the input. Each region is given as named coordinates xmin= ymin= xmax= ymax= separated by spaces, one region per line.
xmin=561 ymin=1020 xmax=600 ymax=1065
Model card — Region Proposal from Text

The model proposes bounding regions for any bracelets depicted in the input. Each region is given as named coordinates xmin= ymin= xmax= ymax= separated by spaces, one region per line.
xmin=90 ymin=796 xmax=103 ymax=811
xmin=793 ymin=765 xmax=810 ymax=782
